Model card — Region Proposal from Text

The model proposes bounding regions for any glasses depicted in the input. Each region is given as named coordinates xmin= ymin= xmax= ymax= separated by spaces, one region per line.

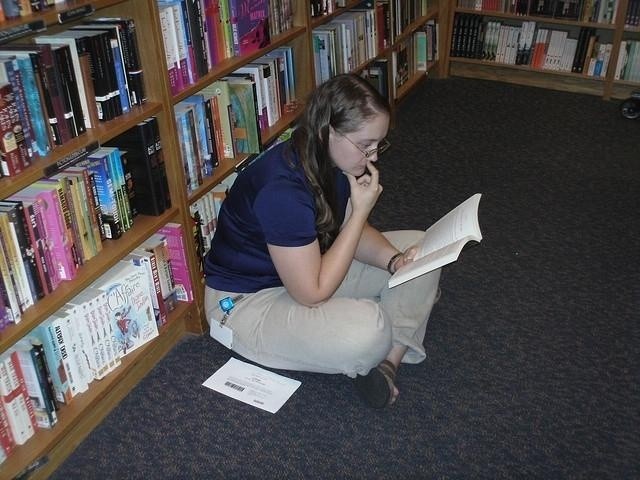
xmin=334 ymin=130 xmax=392 ymax=159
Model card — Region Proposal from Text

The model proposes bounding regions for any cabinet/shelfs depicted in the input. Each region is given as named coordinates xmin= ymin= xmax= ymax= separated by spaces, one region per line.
xmin=0 ymin=1 xmax=209 ymax=479
xmin=443 ymin=0 xmax=640 ymax=101
xmin=152 ymin=0 xmax=449 ymax=325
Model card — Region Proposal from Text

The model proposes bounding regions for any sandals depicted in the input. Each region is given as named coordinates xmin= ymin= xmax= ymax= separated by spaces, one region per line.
xmin=355 ymin=358 xmax=399 ymax=410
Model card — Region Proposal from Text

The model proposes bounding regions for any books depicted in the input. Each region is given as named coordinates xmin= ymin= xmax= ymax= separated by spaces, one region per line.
xmin=154 ymin=0 xmax=297 ymax=96
xmin=580 ymin=0 xmax=619 ymax=24
xmin=388 ymin=192 xmax=485 ymax=290
xmin=535 ymin=29 xmax=578 ymax=73
xmin=0 ymin=114 xmax=172 ymax=331
xmin=615 ymin=40 xmax=640 ymax=83
xmin=189 ymin=154 xmax=252 ymax=263
xmin=309 ymin=0 xmax=345 ymax=19
xmin=0 ymin=219 xmax=194 ymax=465
xmin=449 ymin=11 xmax=488 ymax=59
xmin=577 ymin=26 xmax=613 ymax=79
xmin=624 ymin=1 xmax=640 ymax=26
xmin=171 ymin=44 xmax=298 ymax=198
xmin=505 ymin=0 xmax=530 ymax=15
xmin=554 ymin=0 xmax=582 ymax=21
xmin=0 ymin=0 xmax=94 ymax=27
xmin=352 ymin=37 xmax=412 ymax=99
xmin=312 ymin=3 xmax=378 ymax=86
xmin=456 ymin=0 xmax=483 ymax=11
xmin=375 ymin=0 xmax=427 ymax=46
xmin=487 ymin=17 xmax=536 ymax=67
xmin=0 ymin=16 xmax=146 ymax=176
xmin=413 ymin=19 xmax=440 ymax=71
xmin=481 ymin=1 xmax=506 ymax=14
xmin=529 ymin=1 xmax=556 ymax=18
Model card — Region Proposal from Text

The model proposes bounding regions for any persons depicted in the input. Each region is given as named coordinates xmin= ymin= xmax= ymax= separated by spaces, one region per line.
xmin=202 ymin=73 xmax=442 ymax=406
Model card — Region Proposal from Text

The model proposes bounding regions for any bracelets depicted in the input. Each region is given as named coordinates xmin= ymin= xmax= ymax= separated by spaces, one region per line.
xmin=386 ymin=252 xmax=403 ymax=276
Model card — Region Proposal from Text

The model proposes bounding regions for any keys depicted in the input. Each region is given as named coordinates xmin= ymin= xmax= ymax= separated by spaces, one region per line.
xmin=218 ymin=295 xmax=245 ymax=325
